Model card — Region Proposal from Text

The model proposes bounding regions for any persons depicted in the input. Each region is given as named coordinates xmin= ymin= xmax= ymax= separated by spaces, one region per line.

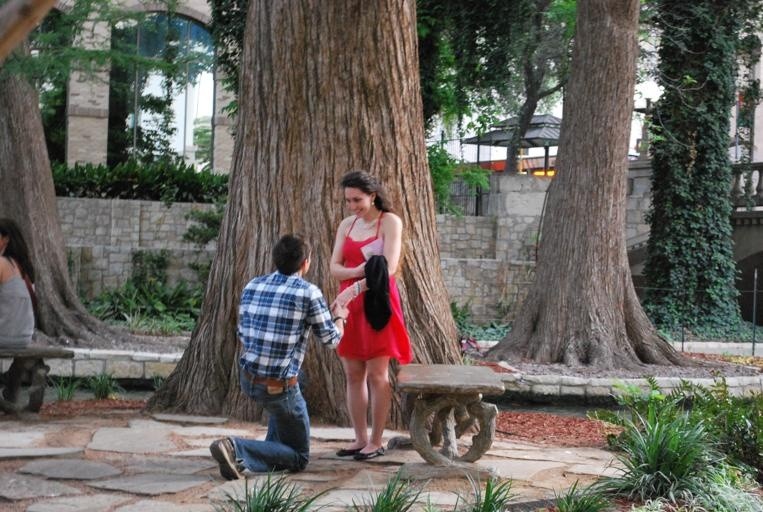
xmin=0 ymin=215 xmax=39 ymax=349
xmin=208 ymin=231 xmax=352 ymax=481
xmin=329 ymin=169 xmax=413 ymax=463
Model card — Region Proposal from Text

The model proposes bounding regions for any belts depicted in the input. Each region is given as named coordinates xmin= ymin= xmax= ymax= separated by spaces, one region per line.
xmin=243 ymin=369 xmax=297 ymax=387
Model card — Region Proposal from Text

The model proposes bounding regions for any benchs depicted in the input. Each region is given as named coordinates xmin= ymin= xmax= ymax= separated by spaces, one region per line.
xmin=397 ymin=364 xmax=505 ymax=482
xmin=0 ymin=348 xmax=75 ymax=415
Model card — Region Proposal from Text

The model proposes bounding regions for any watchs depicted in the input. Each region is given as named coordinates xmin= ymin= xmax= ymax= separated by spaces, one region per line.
xmin=333 ymin=316 xmax=347 ymax=327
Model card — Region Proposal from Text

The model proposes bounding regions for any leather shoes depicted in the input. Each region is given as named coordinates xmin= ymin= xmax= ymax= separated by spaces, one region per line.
xmin=336 ymin=446 xmax=384 ymax=460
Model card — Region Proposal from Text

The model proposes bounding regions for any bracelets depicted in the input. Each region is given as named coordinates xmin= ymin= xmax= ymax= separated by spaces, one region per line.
xmin=355 ymin=280 xmax=361 ymax=297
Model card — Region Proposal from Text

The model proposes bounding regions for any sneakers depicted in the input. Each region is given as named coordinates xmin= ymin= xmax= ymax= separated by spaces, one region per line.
xmin=210 ymin=439 xmax=247 ymax=480
